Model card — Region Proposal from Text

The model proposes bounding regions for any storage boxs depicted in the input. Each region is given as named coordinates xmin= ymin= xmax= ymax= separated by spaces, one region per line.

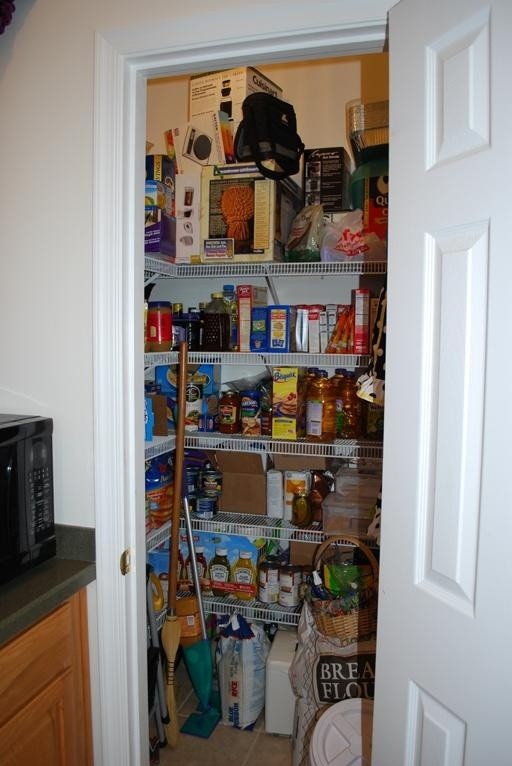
xmin=199 ymin=159 xmax=303 ymax=263
xmin=142 ymin=151 xmax=199 ymax=263
xmin=145 ymin=529 xmax=352 ymax=604
xmin=318 ymin=463 xmax=383 ymax=533
xmin=187 ymin=63 xmax=283 ymax=164
xmin=263 ymin=628 xmax=301 ymax=735
xmin=272 ymin=439 xmax=335 ymax=471
xmin=301 ymin=146 xmax=353 ymax=213
xmin=204 ymin=447 xmax=266 ymax=516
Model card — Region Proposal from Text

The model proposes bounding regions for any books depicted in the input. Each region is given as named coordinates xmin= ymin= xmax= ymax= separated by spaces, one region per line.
xmin=237 ymin=280 xmax=377 ymax=355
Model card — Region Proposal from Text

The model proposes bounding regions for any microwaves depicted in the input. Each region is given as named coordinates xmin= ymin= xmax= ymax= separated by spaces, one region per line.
xmin=1 ymin=413 xmax=58 ymax=579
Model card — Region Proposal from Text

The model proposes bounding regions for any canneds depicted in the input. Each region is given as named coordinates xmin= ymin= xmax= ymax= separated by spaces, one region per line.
xmin=239 ymin=390 xmax=261 ymax=437
xmin=185 ymin=466 xmax=221 ymax=520
xmin=148 ymin=301 xmax=172 ymax=352
xmin=258 ymin=549 xmax=314 ymax=607
xmin=291 ymin=488 xmax=312 ymax=526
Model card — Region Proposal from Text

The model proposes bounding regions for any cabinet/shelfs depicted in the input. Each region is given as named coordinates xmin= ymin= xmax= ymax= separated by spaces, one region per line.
xmin=132 ymin=46 xmax=399 ymax=633
xmin=1 ymin=584 xmax=94 ymax=765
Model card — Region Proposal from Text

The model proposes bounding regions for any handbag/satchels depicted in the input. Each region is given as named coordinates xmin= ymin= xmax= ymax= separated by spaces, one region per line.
xmin=234 ymin=92 xmax=305 ymax=180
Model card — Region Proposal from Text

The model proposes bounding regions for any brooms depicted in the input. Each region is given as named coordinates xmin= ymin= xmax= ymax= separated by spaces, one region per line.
xmin=160 ymin=341 xmax=189 ymax=752
xmin=181 ymin=496 xmax=222 ymax=714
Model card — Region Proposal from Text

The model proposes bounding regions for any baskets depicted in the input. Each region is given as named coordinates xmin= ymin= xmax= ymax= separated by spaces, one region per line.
xmin=305 ymin=537 xmax=379 ymax=640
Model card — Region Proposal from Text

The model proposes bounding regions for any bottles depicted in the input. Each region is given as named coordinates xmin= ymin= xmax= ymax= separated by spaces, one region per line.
xmin=145 ymin=382 xmax=168 ymax=442
xmin=311 ymin=570 xmax=374 ymax=618
xmin=147 ymin=544 xmax=257 ymax=613
xmin=262 ymin=387 xmax=273 ymax=436
xmin=301 ymin=366 xmax=366 ymax=442
xmin=144 ymin=284 xmax=239 ymax=352
